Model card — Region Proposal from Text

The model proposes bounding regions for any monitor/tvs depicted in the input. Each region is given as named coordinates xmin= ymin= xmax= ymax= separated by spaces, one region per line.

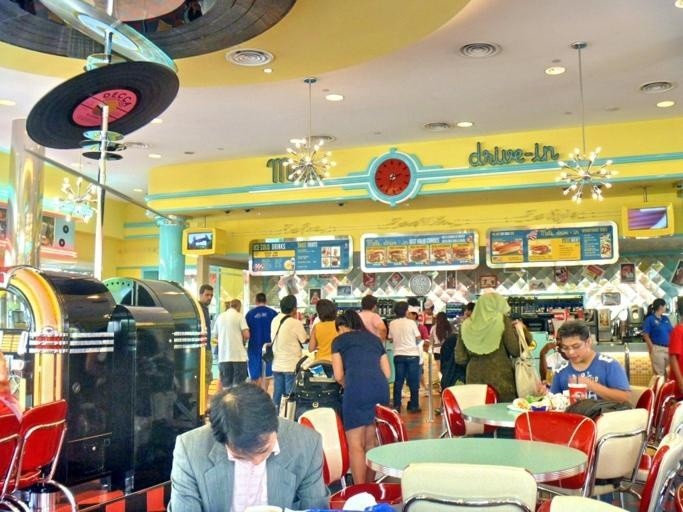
xmin=621 ymin=200 xmax=674 ymax=239
xmin=181 ymin=227 xmax=216 ymax=257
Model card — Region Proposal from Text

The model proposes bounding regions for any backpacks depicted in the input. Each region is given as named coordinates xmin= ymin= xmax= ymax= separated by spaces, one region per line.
xmin=564 ymin=399 xmax=633 ymax=488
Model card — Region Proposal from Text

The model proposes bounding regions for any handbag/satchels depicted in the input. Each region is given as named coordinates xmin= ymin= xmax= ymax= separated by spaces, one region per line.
xmin=261 ymin=343 xmax=274 ymax=362
xmin=278 ymin=393 xmax=297 ymax=421
xmin=515 ymin=357 xmax=542 ymax=398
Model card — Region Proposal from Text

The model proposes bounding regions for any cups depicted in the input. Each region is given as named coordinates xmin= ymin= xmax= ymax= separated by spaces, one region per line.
xmin=568 ymin=383 xmax=588 ymax=405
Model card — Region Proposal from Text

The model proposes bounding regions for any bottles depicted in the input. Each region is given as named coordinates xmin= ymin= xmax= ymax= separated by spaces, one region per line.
xmin=376 ymin=300 xmax=395 ymax=315
xmin=508 ymin=296 xmax=536 ymax=316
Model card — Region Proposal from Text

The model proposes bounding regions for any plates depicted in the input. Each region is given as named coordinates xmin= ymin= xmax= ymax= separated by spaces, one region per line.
xmin=507 ymin=404 xmax=530 ymax=414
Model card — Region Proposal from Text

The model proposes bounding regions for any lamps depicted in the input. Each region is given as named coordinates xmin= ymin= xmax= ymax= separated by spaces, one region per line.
xmin=282 ymin=76 xmax=336 ymax=190
xmin=554 ymin=41 xmax=619 ymax=205
xmin=59 ymin=149 xmax=99 ymax=225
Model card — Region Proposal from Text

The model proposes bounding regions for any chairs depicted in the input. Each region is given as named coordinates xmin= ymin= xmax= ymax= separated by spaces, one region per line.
xmin=0 ymin=398 xmax=83 ymax=512
xmin=0 ymin=411 xmax=34 ymax=512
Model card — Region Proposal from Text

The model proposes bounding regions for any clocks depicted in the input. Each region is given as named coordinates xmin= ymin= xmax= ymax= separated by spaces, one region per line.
xmin=365 ymin=151 xmax=420 ymax=205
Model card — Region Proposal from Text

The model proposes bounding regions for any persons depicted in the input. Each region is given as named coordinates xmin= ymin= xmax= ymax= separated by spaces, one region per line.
xmin=199 ymin=283 xmax=339 ymax=405
xmin=332 ymin=307 xmax=391 ymax=484
xmin=166 ymin=381 xmax=331 ymax=511
xmin=359 ymin=294 xmax=476 ymax=411
xmin=454 ymin=290 xmax=532 ymax=403
xmin=537 ymin=320 xmax=633 ymax=407
xmin=641 ymin=296 xmax=683 ymax=402
